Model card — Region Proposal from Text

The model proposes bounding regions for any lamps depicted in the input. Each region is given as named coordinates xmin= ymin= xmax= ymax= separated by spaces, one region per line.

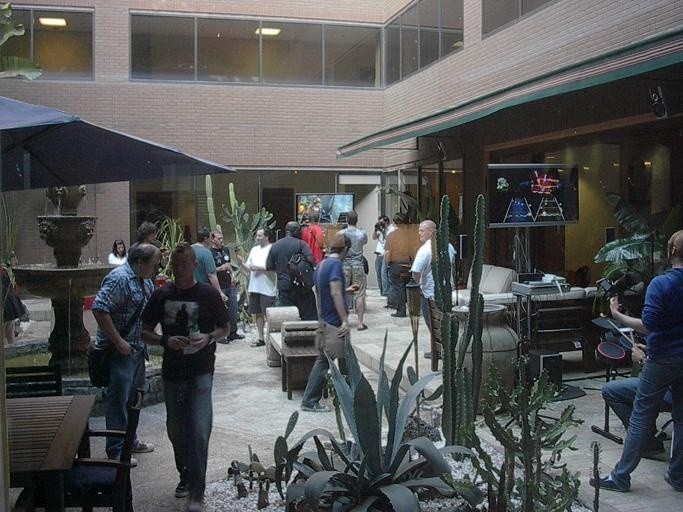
xmin=647 ymin=87 xmax=671 ymax=121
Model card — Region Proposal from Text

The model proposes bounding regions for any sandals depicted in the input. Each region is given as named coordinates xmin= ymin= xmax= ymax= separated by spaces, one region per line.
xmin=251 ymin=340 xmax=264 ymax=347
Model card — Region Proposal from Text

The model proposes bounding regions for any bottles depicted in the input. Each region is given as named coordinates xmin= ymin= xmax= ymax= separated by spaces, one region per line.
xmin=188 ymin=321 xmax=199 ymax=347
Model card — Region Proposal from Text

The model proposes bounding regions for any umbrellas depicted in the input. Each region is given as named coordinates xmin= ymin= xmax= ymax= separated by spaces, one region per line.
xmin=0 ymin=91 xmax=242 ymax=193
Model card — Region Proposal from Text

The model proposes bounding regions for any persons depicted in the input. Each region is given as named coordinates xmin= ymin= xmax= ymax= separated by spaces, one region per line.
xmin=90 ymin=242 xmax=161 ymax=468
xmin=600 ymin=294 xmax=673 ymax=459
xmin=0 ymin=268 xmax=25 ymax=346
xmin=589 ymin=230 xmax=682 ymax=493
xmin=410 ymin=223 xmax=457 ymax=360
xmin=138 ymin=242 xmax=232 ymax=511
xmin=297 ymin=233 xmax=360 ymax=413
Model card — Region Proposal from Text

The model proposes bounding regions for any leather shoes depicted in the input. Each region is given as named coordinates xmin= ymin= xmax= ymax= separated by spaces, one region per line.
xmin=424 ymin=351 xmax=440 ymax=359
xmin=233 ymin=334 xmax=244 ymax=339
xmin=642 ymin=439 xmax=665 ymax=459
xmin=217 ymin=339 xmax=228 ymax=344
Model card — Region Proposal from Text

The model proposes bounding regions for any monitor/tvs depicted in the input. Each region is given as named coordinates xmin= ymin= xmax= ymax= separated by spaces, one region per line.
xmin=294 ymin=192 xmax=355 ymax=228
xmin=486 ymin=164 xmax=580 ymax=229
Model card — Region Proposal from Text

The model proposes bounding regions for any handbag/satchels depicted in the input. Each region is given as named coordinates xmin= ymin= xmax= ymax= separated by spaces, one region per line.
xmin=87 ymin=347 xmax=114 ymax=389
xmin=315 ymin=318 xmax=345 ymax=358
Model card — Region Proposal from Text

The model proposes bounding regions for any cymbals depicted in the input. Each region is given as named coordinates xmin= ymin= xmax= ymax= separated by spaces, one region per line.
xmin=591 ymin=317 xmax=621 ymax=329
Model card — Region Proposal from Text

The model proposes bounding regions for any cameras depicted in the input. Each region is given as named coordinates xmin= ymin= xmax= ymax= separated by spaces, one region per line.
xmin=375 ymin=222 xmax=385 ymax=229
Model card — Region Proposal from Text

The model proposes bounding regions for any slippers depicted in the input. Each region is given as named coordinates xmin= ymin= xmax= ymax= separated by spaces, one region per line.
xmin=358 ymin=324 xmax=367 ymax=330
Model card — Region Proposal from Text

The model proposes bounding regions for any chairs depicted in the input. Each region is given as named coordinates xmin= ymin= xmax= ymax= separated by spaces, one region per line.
xmin=424 ymin=296 xmax=459 ymax=371
xmin=451 ymin=263 xmax=646 ymax=373
xmin=28 ymin=387 xmax=145 ymax=512
xmin=282 ymin=327 xmax=328 ymax=400
xmin=4 ymin=366 xmax=63 ymax=399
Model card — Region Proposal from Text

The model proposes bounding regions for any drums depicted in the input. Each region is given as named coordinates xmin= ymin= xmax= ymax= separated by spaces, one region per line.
xmin=597 ymin=342 xmax=626 ymax=361
xmin=620 ymin=334 xmax=641 ymax=350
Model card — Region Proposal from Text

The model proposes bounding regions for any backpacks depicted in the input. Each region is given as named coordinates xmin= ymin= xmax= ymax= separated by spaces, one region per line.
xmin=286 ymin=240 xmax=315 ymax=297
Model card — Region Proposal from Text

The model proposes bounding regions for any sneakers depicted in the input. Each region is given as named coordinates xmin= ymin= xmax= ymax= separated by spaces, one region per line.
xmin=175 ymin=481 xmax=189 ymax=497
xmin=589 ymin=474 xmax=627 ymax=492
xmin=663 ymin=471 xmax=682 ymax=492
xmin=131 ymin=441 xmax=154 ymax=453
xmin=111 ymin=455 xmax=138 ymax=467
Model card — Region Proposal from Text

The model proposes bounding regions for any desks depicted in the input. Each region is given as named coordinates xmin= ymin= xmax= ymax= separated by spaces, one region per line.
xmin=6 ymin=395 xmax=97 ymax=510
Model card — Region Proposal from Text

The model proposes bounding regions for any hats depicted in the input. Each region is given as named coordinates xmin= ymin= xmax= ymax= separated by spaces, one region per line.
xmin=329 ymin=234 xmax=350 ymax=252
